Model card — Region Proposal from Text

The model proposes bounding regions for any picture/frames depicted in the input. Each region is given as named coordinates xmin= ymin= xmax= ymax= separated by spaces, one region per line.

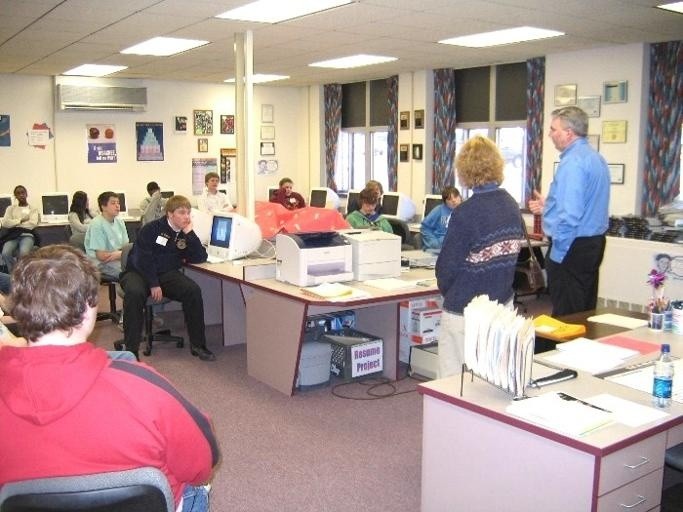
xmin=607 ymin=164 xmax=625 ymax=184
xmin=193 ymin=110 xmax=213 ymax=136
xmin=198 ymin=138 xmax=208 ymax=152
xmin=601 ymin=120 xmax=627 ymax=144
xmin=260 ymin=142 xmax=275 ymax=155
xmin=586 ymin=134 xmax=600 ymax=152
xmin=174 ymin=115 xmax=188 ymax=133
xmin=220 ymin=115 xmax=235 ymax=134
xmin=552 ymin=161 xmax=560 ymax=175
xmin=261 ymin=103 xmax=273 ymax=123
xmin=576 ymin=95 xmax=601 ymax=118
xmin=260 ymin=126 xmax=275 ymax=140
xmin=602 ymin=80 xmax=627 ymax=104
xmin=554 ymin=84 xmax=578 ymax=107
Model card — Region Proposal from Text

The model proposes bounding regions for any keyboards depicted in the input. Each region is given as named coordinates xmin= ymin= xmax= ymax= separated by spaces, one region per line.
xmin=206 ymin=255 xmax=224 ymax=263
xmin=40 ymin=215 xmax=67 ymax=224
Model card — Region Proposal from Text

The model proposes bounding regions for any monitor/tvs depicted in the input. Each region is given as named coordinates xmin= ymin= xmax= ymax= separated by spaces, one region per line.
xmin=42 ymin=195 xmax=68 ymax=215
xmin=118 ymin=193 xmax=126 ymax=212
xmin=268 ymin=189 xmax=278 ymax=202
xmin=309 ymin=187 xmax=338 ymax=208
xmin=421 ymin=195 xmax=442 ymax=220
xmin=218 ymin=190 xmax=226 ymax=194
xmin=0 ymin=198 xmax=11 ymax=217
xmin=346 ymin=192 xmax=361 ymax=215
xmin=207 ymin=211 xmax=262 ymax=260
xmin=380 ymin=192 xmax=416 ymax=221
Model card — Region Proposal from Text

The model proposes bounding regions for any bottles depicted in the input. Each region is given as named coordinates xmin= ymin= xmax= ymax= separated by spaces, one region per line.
xmin=650 ymin=344 xmax=673 ymax=410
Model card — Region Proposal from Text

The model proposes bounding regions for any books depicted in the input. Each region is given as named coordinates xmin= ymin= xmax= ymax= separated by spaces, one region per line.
xmin=303 ymin=283 xmax=352 ymax=299
xmin=546 ymin=333 xmax=660 ymax=376
xmin=534 ymin=315 xmax=587 ymax=340
xmin=532 ymin=362 xmax=577 ymax=386
xmin=508 ymin=392 xmax=615 ymax=439
xmin=588 ymin=313 xmax=648 ymax=329
xmin=114 ymin=193 xmax=129 ymax=221
xmin=40 ymin=193 xmax=71 ymax=225
xmin=463 ymin=294 xmax=535 ymax=400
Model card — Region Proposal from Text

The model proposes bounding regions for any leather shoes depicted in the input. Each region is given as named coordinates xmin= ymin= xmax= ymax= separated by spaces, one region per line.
xmin=190 ymin=344 xmax=215 ymax=360
xmin=125 ymin=348 xmax=139 ymax=362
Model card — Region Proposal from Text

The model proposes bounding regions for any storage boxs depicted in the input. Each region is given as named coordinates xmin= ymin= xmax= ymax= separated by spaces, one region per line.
xmin=400 ymin=297 xmax=442 ymax=365
xmin=408 ymin=342 xmax=438 ymax=382
xmin=411 ymin=306 xmax=442 ymax=345
xmin=320 ymin=328 xmax=383 ymax=382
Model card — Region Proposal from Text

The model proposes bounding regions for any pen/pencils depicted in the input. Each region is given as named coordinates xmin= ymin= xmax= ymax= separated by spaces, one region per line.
xmin=646 ymin=296 xmax=672 ymax=315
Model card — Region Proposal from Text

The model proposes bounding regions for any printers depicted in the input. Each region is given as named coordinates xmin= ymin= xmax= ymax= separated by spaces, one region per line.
xmin=275 ymin=232 xmax=353 ymax=287
xmin=336 ymin=228 xmax=401 ymax=281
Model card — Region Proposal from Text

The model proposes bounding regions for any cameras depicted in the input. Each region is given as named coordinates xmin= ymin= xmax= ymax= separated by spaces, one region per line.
xmin=160 ymin=192 xmax=173 ymax=198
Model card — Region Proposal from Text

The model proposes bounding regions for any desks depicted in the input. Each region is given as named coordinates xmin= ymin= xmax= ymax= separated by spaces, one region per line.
xmin=182 ymin=251 xmax=443 ymax=397
xmin=113 ymin=242 xmax=184 ymax=356
xmin=512 ymin=238 xmax=551 ymax=304
xmin=0 ymin=214 xmax=141 ymax=248
xmin=417 ymin=325 xmax=683 ymax=512
xmin=405 ymin=222 xmax=423 ymax=233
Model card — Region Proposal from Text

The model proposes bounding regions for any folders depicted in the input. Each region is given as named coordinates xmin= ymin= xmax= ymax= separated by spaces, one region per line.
xmin=532 ymin=358 xmax=577 ymax=388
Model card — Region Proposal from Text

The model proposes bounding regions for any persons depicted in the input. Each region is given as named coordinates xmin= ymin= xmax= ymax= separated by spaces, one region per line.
xmin=85 ymin=192 xmax=162 ymax=331
xmin=270 ymin=178 xmax=305 ymax=209
xmin=656 ymin=254 xmax=670 ymax=273
xmin=421 ymin=188 xmax=462 ymax=249
xmin=199 ymin=173 xmax=233 ymax=216
xmin=2 ymin=186 xmax=38 ymax=273
xmin=345 ymin=189 xmax=392 ymax=234
xmin=120 ymin=196 xmax=217 ymax=362
xmin=434 ymin=135 xmax=532 ymax=377
xmin=69 ymin=192 xmax=94 ymax=236
xmin=139 ymin=182 xmax=170 ymax=226
xmin=527 ymin=107 xmax=611 ymax=317
xmin=366 ymin=180 xmax=382 ymax=201
xmin=258 ymin=160 xmax=268 ymax=178
xmin=0 ymin=245 xmax=222 ymax=508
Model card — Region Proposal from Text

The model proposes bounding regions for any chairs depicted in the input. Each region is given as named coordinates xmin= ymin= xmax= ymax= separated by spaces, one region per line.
xmin=12 ymin=246 xmax=39 ymax=257
xmin=64 ymin=224 xmax=73 ymax=242
xmin=413 ymin=233 xmax=425 ymax=249
xmin=0 ymin=468 xmax=175 ymax=512
xmin=70 ymin=233 xmax=120 ymax=324
xmin=387 ymin=218 xmax=411 ymax=245
